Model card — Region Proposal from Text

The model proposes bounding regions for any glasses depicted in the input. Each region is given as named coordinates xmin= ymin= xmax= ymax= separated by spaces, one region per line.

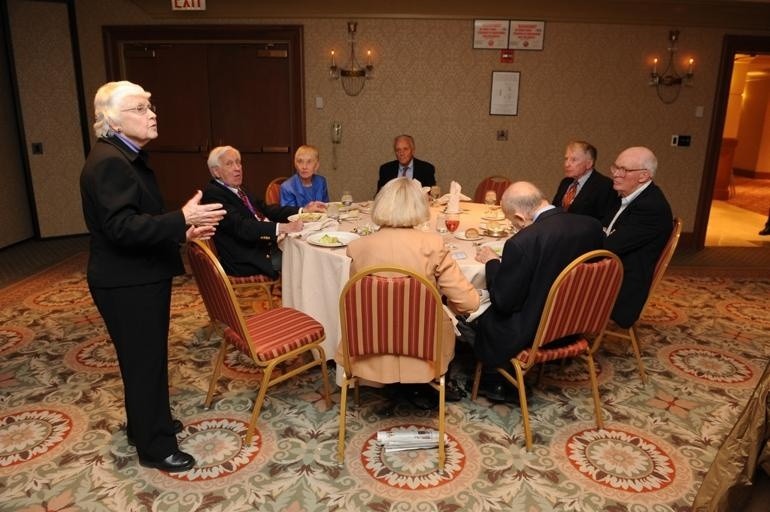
xmin=609 ymin=162 xmax=647 ymax=178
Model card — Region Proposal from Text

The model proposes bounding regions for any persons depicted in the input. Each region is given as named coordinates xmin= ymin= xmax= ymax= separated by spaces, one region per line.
xmin=551 ymin=139 xmax=621 ymax=224
xmin=374 ymin=132 xmax=436 ymax=200
xmin=79 ymin=81 xmax=227 ymax=473
xmin=280 ymin=144 xmax=329 ymax=212
xmin=604 ymin=146 xmax=673 ymax=329
xmin=336 ymin=175 xmax=480 ymax=401
xmin=200 ymin=145 xmax=328 ymax=281
xmin=466 ymin=180 xmax=606 ymax=402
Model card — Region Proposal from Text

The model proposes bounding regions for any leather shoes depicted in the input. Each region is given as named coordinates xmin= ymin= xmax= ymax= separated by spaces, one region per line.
xmin=139 ymin=448 xmax=195 ymax=473
xmin=465 ymin=379 xmax=506 ymax=401
xmin=171 ymin=419 xmax=182 ymax=432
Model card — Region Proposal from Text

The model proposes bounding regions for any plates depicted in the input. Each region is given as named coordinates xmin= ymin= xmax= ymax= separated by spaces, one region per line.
xmin=481 ymin=241 xmax=508 ymax=256
xmin=307 ymin=232 xmax=361 ymax=248
xmin=288 ymin=213 xmax=327 ymax=223
xmin=325 ymin=201 xmax=357 ymax=211
xmin=455 ymin=231 xmax=483 ymax=241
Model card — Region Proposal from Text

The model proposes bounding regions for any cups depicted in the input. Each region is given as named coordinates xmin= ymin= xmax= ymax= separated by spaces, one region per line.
xmin=437 ymin=213 xmax=449 ymax=234
xmin=445 ymin=213 xmax=460 ymax=251
xmin=486 ymin=190 xmax=496 ymax=214
xmin=431 ymin=187 xmax=440 ymax=205
xmin=342 ymin=191 xmax=353 ymax=214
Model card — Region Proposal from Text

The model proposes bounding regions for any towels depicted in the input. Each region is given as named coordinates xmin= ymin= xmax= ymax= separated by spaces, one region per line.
xmin=433 ymin=180 xmax=472 ymax=212
xmin=298 ymin=218 xmax=339 ymax=242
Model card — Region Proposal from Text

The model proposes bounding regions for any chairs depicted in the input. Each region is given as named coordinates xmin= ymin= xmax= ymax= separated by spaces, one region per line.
xmin=201 ymin=240 xmax=281 ymax=341
xmin=470 ymin=250 xmax=624 ymax=450
xmin=336 ymin=263 xmax=446 ymax=470
xmin=186 ymin=238 xmax=332 ymax=445
xmin=264 ymin=177 xmax=288 ymax=205
xmin=603 ymin=215 xmax=683 ymax=384
xmin=473 ymin=175 xmax=512 ymax=205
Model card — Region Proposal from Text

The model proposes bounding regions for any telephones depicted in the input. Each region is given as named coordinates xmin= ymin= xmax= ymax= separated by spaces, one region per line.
xmin=332 ymin=121 xmax=342 ymax=170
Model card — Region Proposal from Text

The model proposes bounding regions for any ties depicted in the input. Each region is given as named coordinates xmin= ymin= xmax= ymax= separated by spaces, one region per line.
xmin=402 ymin=167 xmax=409 ymax=177
xmin=561 ymin=180 xmax=579 ymax=208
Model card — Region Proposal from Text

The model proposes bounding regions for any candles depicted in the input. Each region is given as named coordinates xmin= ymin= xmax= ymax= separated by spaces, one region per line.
xmin=651 ymin=57 xmax=659 ymax=72
xmin=367 ymin=50 xmax=371 ymax=65
xmin=331 ymin=51 xmax=336 ymax=66
xmin=687 ymin=58 xmax=694 ymax=74
